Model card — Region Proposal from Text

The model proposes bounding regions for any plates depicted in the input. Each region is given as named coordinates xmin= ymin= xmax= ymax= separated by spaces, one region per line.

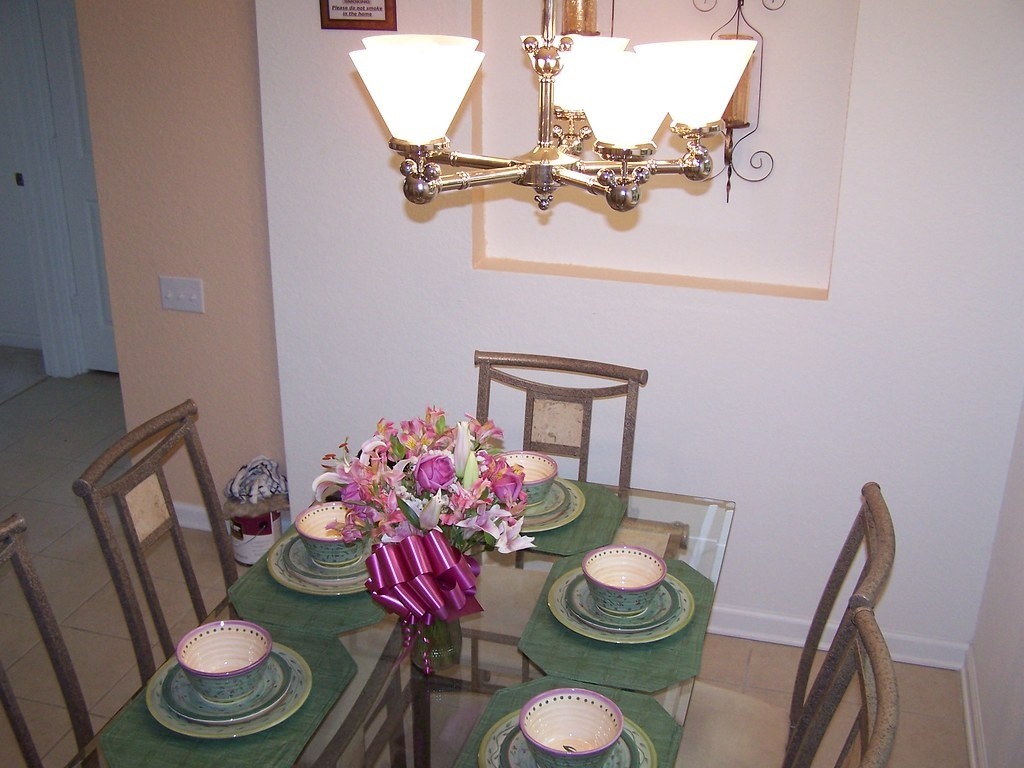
xmin=146 ymin=644 xmax=311 ymax=738
xmin=285 ymin=536 xmax=379 ymax=585
xmin=523 ymin=480 xmax=571 ymax=525
xmin=478 ymin=709 xmax=658 ymax=768
xmin=162 ymin=652 xmax=294 ymax=724
xmin=267 ymin=530 xmax=370 ymax=594
xmin=547 ymin=568 xmax=695 ymax=644
xmin=519 ymin=477 xmax=585 ymax=533
xmin=565 ymin=574 xmax=679 ymax=633
xmin=501 ymin=724 xmax=639 ymax=768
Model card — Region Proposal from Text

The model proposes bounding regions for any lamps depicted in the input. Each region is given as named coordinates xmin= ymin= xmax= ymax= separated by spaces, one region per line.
xmin=343 ymin=0 xmax=758 ymax=217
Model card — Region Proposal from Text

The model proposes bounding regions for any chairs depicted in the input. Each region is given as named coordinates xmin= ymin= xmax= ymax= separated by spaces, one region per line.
xmin=409 ymin=348 xmax=652 ymax=768
xmin=72 ymin=396 xmax=410 ymax=768
xmin=790 ymin=606 xmax=908 ymax=768
xmin=0 ymin=510 xmax=101 ymax=768
xmin=673 ymin=479 xmax=897 ymax=768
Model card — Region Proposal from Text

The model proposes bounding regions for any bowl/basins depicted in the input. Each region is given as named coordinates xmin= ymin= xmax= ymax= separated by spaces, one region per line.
xmin=520 ymin=688 xmax=624 ymax=768
xmin=582 ymin=545 xmax=667 ymax=616
xmin=176 ymin=620 xmax=273 ymax=704
xmin=295 ymin=502 xmax=366 ymax=570
xmin=501 ymin=451 xmax=558 ymax=507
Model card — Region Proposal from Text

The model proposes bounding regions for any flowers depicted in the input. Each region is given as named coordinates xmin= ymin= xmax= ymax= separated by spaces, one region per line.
xmin=309 ymin=390 xmax=529 ymax=569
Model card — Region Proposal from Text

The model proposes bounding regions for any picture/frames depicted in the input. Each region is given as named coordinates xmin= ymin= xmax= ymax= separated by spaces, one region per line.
xmin=319 ymin=0 xmax=397 ymax=32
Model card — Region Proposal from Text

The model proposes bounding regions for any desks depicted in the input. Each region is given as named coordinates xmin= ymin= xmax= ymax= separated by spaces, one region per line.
xmin=64 ymin=477 xmax=735 ymax=768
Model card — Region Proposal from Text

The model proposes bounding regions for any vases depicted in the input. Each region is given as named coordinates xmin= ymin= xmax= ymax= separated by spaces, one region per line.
xmin=399 ymin=547 xmax=477 ymax=670
